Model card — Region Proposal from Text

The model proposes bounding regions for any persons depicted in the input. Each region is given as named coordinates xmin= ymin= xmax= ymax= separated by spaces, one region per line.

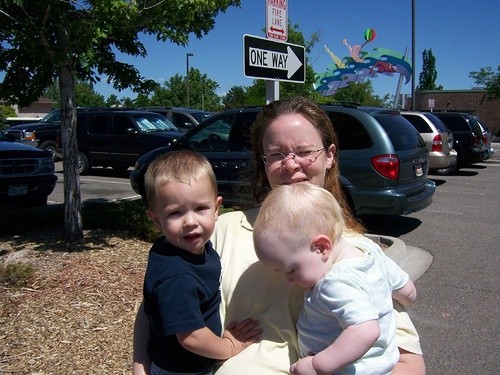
xmin=132 ymin=95 xmax=427 ymax=375
xmin=143 ymin=152 xmax=262 ymax=375
xmin=254 ymin=182 xmax=417 ymax=375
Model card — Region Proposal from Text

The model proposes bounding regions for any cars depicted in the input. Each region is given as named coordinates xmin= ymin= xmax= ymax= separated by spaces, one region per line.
xmin=130 ymin=101 xmax=437 ymax=220
xmin=400 ymin=111 xmax=491 ymax=173
xmin=0 ymin=136 xmax=58 ymax=208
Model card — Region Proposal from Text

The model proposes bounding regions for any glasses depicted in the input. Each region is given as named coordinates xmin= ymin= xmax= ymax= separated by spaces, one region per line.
xmin=261 ymin=147 xmax=325 ymax=166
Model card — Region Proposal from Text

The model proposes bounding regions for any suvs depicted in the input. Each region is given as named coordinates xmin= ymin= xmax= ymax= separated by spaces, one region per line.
xmin=146 ymin=109 xmax=231 ymax=142
xmin=76 ymin=110 xmax=187 ymax=176
xmin=3 ymin=107 xmax=102 ymax=161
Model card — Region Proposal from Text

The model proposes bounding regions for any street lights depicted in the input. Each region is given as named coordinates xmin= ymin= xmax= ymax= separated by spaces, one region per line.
xmin=185 ymin=53 xmax=194 ymax=108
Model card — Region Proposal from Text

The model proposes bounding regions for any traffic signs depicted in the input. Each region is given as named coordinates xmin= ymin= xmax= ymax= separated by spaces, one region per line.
xmin=243 ymin=34 xmax=306 ymax=84
xmin=264 ymin=0 xmax=289 ymax=44
